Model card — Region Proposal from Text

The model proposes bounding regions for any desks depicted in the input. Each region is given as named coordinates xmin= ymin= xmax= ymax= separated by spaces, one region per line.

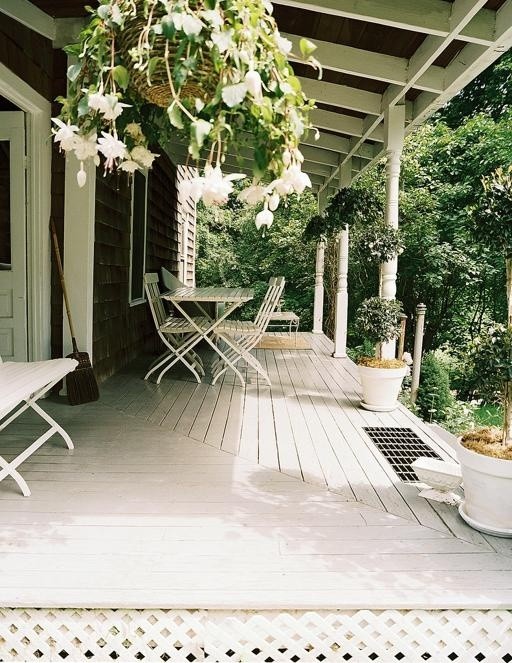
xmin=259 ymin=310 xmax=300 ymax=347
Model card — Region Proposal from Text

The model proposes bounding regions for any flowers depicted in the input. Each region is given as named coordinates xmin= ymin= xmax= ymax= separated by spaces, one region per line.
xmin=49 ymin=0 xmax=323 ymax=239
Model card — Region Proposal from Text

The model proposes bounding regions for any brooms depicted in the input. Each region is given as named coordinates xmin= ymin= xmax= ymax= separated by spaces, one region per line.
xmin=49 ymin=215 xmax=100 ymax=407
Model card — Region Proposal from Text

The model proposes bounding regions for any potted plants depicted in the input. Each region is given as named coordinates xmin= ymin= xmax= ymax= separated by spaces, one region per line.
xmin=353 ymin=222 xmax=407 ymax=412
xmin=449 ymin=166 xmax=512 ymax=540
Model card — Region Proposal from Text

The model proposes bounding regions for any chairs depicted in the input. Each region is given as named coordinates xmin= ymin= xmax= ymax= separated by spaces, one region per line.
xmin=141 ymin=272 xmax=286 ymax=387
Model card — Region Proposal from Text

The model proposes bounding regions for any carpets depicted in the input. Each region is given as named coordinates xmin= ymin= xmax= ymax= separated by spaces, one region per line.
xmin=244 ymin=335 xmax=312 ymax=350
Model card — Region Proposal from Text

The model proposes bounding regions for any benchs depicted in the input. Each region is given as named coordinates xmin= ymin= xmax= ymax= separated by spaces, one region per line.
xmin=0 ymin=352 xmax=79 ymax=498
